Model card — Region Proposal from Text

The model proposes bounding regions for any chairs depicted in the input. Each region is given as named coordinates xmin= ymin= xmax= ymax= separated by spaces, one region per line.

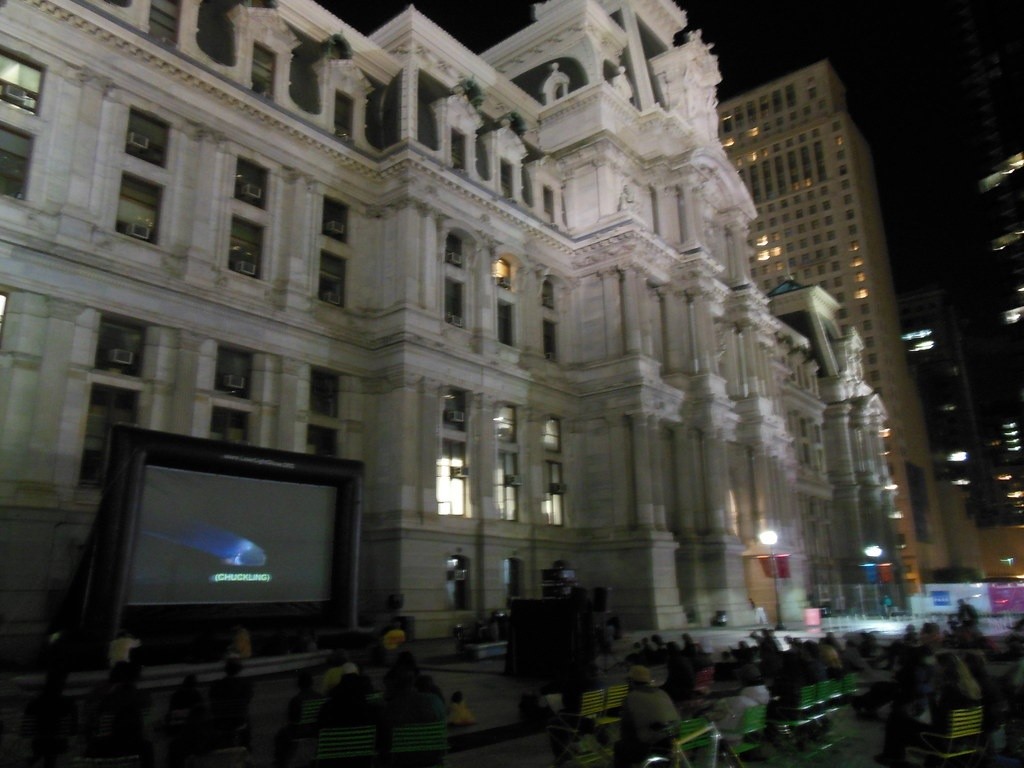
xmin=389 ymin=719 xmax=451 ymax=768
xmin=171 ymin=696 xmax=250 ymax=747
xmin=545 ymin=680 xmax=655 ymax=768
xmin=293 ymin=691 xmax=384 ymax=742
xmin=17 ymin=699 xmax=156 ymax=768
xmin=905 ymin=706 xmax=983 ymax=768
xmin=664 ymin=705 xmax=767 ymax=768
xmin=768 ymin=672 xmax=860 ymax=760
xmin=306 ymin=725 xmax=380 ymax=768
xmin=649 ymin=663 xmax=669 ymax=687
xmin=678 ymin=666 xmax=715 ymax=716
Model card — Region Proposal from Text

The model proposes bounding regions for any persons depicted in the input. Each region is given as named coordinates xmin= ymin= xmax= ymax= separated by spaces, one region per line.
xmin=549 ymin=604 xmax=1024 ymax=768
xmin=19 ymin=623 xmax=449 ymax=768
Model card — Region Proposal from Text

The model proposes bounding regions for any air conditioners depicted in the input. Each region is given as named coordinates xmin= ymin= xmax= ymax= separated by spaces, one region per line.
xmin=339 ymin=135 xmax=354 ymax=145
xmin=126 ymin=224 xmax=150 ymax=239
xmin=550 ymin=483 xmax=567 ymax=493
xmin=498 ymin=277 xmax=510 ymax=288
xmin=325 ymin=292 xmax=340 ymax=305
xmin=126 ymin=131 xmax=148 ymax=149
xmin=236 ymin=261 xmax=256 ymax=275
xmin=1 ymin=85 xmax=26 ymax=101
xmin=454 ymin=169 xmax=469 ymax=177
xmin=446 ymin=411 xmax=464 ymax=422
xmin=448 ymin=315 xmax=463 ymax=327
xmin=546 ymin=352 xmax=554 ymax=360
xmin=451 ymin=467 xmax=468 ymax=479
xmin=447 ymin=570 xmax=465 ymax=581
xmin=241 ymin=183 xmax=261 ymax=199
xmin=222 ymin=375 xmax=245 ymax=389
xmin=259 ymin=92 xmax=274 ymax=101
xmin=159 ymin=38 xmax=177 ymax=49
xmin=507 ymin=198 xmax=517 ymax=204
xmin=106 ymin=349 xmax=133 ymax=364
xmin=507 ymin=474 xmax=523 ymax=486
xmin=326 ymin=221 xmax=344 ymax=234
xmin=448 ymin=252 xmax=462 ymax=264
xmin=543 ymin=296 xmax=553 ymax=307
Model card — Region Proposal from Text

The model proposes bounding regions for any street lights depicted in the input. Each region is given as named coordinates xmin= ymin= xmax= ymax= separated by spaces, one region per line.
xmin=758 ymin=530 xmax=787 ymax=631
xmin=864 ymin=546 xmax=893 ymax=621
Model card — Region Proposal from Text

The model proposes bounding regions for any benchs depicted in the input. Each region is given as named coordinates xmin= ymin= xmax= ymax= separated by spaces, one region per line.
xmin=465 ymin=641 xmax=508 ymax=660
xmin=843 ymin=632 xmax=903 ymax=646
xmin=11 ymin=650 xmax=333 ymax=699
